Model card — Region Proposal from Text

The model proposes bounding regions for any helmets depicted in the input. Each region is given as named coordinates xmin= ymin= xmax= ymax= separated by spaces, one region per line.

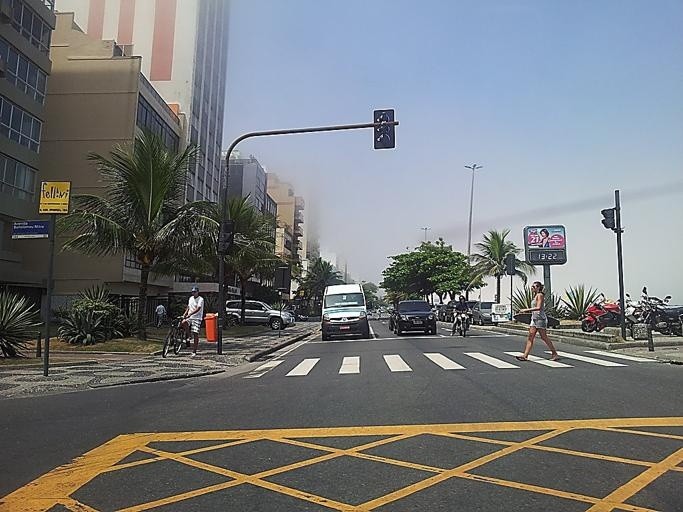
xmin=457 ymin=294 xmax=465 ymax=302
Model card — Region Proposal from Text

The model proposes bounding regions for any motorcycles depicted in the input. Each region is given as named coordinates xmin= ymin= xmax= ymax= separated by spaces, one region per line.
xmin=450 ymin=306 xmax=472 ymax=337
xmin=579 ymin=287 xmax=682 ymax=337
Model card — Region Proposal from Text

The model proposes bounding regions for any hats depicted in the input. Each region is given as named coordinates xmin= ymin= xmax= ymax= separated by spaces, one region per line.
xmin=191 ymin=286 xmax=200 ymax=293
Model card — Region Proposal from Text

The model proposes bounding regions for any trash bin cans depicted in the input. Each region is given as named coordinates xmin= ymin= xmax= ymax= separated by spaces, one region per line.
xmin=203 ymin=312 xmax=219 ymax=342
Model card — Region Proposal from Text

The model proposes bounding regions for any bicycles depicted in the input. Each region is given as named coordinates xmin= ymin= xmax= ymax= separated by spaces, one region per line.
xmin=161 ymin=314 xmax=186 ymax=357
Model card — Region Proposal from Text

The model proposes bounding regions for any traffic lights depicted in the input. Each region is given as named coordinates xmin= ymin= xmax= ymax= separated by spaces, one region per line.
xmin=599 ymin=207 xmax=615 ymax=229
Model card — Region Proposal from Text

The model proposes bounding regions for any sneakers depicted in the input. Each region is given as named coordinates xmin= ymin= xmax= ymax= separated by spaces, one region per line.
xmin=189 ymin=351 xmax=197 ymax=360
xmin=515 ymin=352 xmax=560 ymax=362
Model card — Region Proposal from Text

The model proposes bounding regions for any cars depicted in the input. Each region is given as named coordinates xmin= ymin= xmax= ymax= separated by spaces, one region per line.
xmin=366 ymin=304 xmax=394 ymax=316
xmin=431 ymin=301 xmax=510 ymax=327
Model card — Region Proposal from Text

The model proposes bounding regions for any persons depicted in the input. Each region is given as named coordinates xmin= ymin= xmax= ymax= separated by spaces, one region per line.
xmin=451 ymin=295 xmax=469 ymax=336
xmin=539 ymin=229 xmax=550 ymax=247
xmin=181 ymin=287 xmax=204 ymax=359
xmin=155 ymin=302 xmax=167 ymax=328
xmin=516 ymin=282 xmax=559 ymax=361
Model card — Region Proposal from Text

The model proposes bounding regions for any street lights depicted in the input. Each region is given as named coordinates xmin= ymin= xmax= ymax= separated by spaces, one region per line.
xmin=419 ymin=224 xmax=432 ymax=241
xmin=462 ymin=163 xmax=484 ymax=266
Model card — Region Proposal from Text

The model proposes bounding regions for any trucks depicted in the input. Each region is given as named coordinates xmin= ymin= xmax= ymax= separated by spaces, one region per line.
xmin=319 ymin=282 xmax=368 ymax=341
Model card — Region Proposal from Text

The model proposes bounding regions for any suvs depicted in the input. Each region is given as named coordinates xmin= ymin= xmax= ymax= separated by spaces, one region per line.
xmin=225 ymin=300 xmax=293 ymax=331
